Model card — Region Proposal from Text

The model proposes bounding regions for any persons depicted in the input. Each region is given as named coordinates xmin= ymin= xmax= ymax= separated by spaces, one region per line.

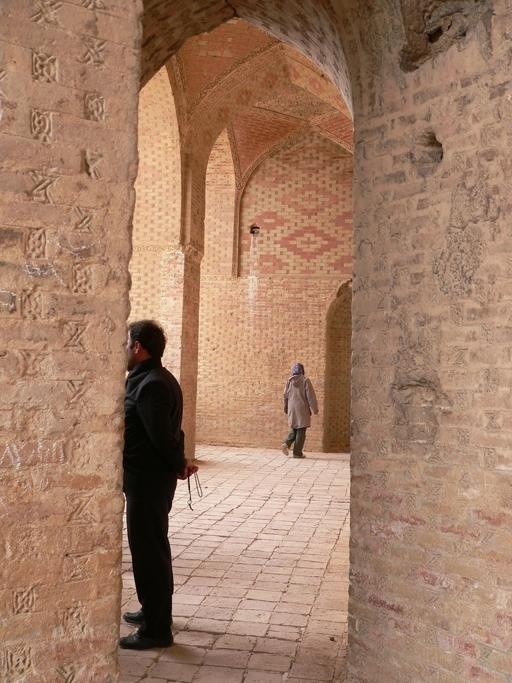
xmin=118 ymin=318 xmax=199 ymax=650
xmin=280 ymin=363 xmax=319 ymax=459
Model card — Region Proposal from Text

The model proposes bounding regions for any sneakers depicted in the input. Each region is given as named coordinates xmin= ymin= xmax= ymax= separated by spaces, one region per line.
xmin=293 ymin=453 xmax=306 ymax=459
xmin=281 ymin=443 xmax=290 ymax=456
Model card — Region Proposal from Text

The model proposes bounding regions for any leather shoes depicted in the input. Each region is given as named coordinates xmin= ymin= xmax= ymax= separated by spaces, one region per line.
xmin=119 ymin=632 xmax=174 ymax=650
xmin=123 ymin=608 xmax=147 ymax=625
